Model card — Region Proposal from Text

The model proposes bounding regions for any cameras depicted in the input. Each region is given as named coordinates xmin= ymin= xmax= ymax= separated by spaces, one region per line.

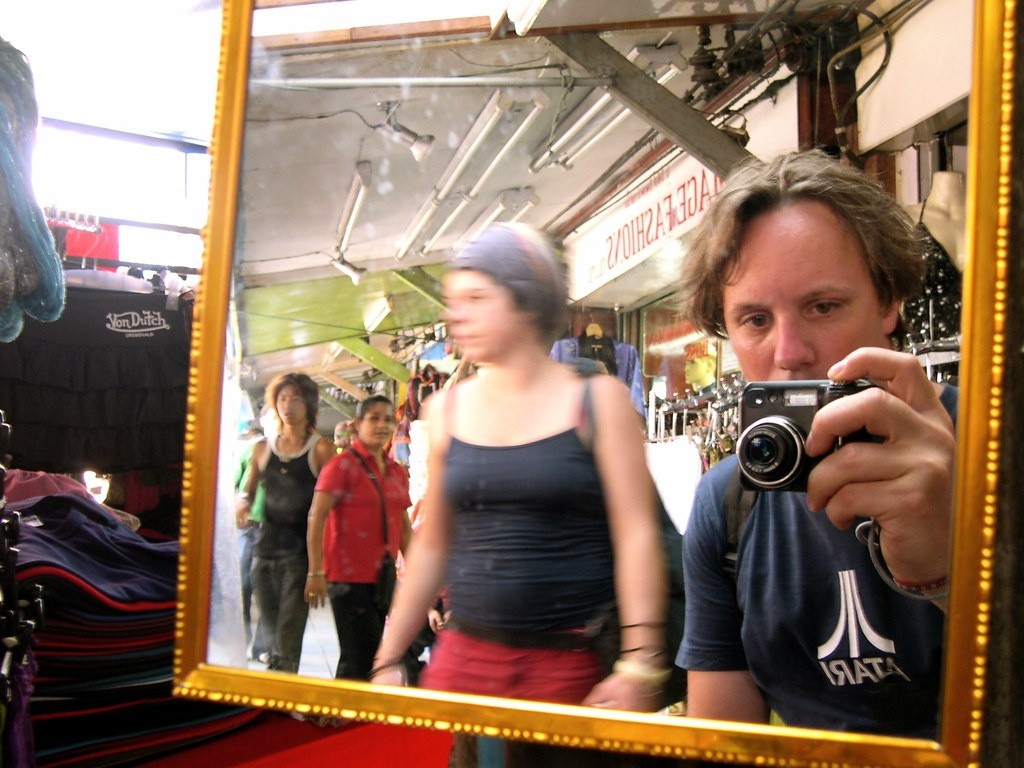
xmin=737 ymin=379 xmax=882 ymax=493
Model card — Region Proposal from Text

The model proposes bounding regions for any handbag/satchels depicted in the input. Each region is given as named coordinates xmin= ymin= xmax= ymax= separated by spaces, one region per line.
xmin=586 ymin=528 xmax=687 ymax=710
xmin=378 ymin=556 xmax=397 ymax=592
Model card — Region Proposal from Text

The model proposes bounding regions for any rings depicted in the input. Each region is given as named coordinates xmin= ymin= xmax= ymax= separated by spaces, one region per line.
xmin=308 ymin=593 xmax=316 ymax=597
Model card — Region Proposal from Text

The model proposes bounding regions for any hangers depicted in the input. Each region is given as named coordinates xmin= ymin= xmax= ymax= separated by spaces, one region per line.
xmin=584 ymin=310 xmax=605 ymax=348
xmin=47 ymin=207 xmax=191 ymax=311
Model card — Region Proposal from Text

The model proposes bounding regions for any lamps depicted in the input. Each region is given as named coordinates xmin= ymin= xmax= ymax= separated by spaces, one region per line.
xmin=392 ymin=40 xmax=690 ymax=261
xmin=329 ymin=245 xmax=367 ymax=287
xmin=381 ymin=119 xmax=436 ymax=163
xmin=335 ymin=161 xmax=373 ymax=254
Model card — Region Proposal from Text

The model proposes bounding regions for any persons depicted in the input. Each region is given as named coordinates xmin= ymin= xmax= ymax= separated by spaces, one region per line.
xmin=237 ymin=151 xmax=957 ymax=723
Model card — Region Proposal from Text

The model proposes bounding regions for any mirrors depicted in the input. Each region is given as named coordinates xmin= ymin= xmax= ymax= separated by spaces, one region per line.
xmin=639 ymin=287 xmax=723 ymax=401
xmin=172 ymin=0 xmax=1018 ymax=768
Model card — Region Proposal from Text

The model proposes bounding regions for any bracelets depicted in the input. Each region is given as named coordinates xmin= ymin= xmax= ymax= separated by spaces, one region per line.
xmin=893 ymin=576 xmax=947 ymax=592
xmin=620 ymin=645 xmax=657 ymax=653
xmin=614 ymin=661 xmax=671 ymax=683
xmin=308 ymin=572 xmax=325 ymax=577
xmin=370 ymin=660 xmax=402 ymax=676
xmin=620 ymin=621 xmax=667 ymax=629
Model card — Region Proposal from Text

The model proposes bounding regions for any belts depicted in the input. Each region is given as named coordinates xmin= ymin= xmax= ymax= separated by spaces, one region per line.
xmin=446 ymin=617 xmax=594 ymax=652
